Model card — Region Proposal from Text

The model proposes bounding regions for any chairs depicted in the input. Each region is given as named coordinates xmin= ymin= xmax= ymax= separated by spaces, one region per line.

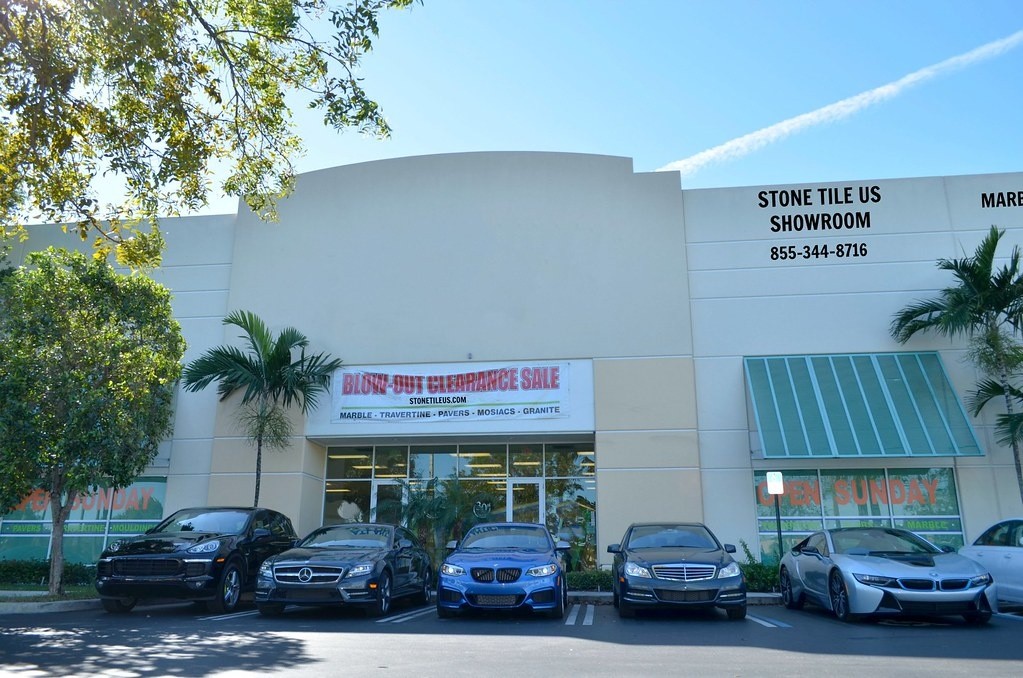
xmin=634 ymin=535 xmax=653 ymax=548
xmin=676 ymin=535 xmax=698 ymax=545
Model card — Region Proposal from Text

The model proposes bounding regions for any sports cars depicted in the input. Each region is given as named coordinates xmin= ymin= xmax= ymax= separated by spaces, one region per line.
xmin=779 ymin=526 xmax=999 ymax=628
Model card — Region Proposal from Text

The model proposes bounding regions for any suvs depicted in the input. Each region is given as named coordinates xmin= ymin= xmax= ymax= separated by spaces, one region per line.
xmin=95 ymin=506 xmax=301 ymax=615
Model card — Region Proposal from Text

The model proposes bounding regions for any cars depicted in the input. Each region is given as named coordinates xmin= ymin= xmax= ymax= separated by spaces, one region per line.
xmin=607 ymin=522 xmax=747 ymax=620
xmin=254 ymin=522 xmax=435 ymax=617
xmin=436 ymin=522 xmax=572 ymax=618
xmin=553 ymin=524 xmax=588 ymax=546
xmin=956 ymin=517 xmax=1023 ymax=604
